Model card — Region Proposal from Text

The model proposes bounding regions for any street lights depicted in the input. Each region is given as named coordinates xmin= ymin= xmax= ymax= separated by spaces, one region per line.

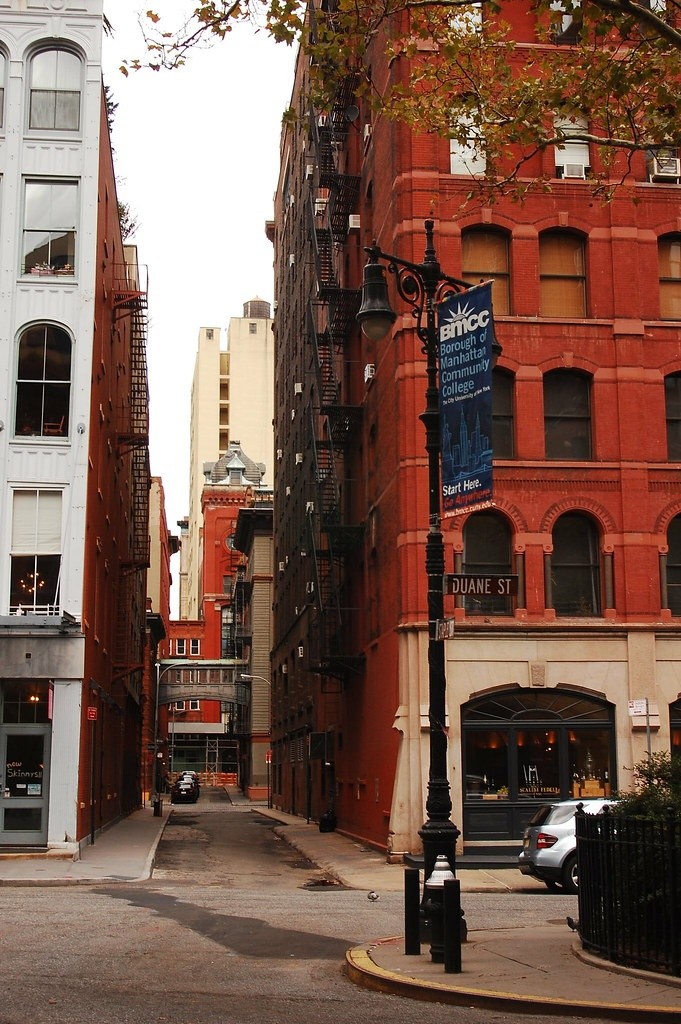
xmin=358 ymin=218 xmax=504 ymax=968
xmin=151 ymin=659 xmax=201 ymax=808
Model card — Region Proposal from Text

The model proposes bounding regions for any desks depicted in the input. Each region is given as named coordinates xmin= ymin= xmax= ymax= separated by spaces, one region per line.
xmin=45 ymin=423 xmax=60 ymax=429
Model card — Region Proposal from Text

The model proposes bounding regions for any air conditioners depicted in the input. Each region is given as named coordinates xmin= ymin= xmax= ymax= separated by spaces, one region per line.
xmin=282 ymin=665 xmax=288 ymax=673
xmin=364 ymin=124 xmax=372 ymax=144
xmin=286 ymin=486 xmax=290 ymax=496
xmin=315 ymin=199 xmax=328 ymax=216
xmin=364 ymin=364 xmax=375 ymax=383
xmin=347 ymin=215 xmax=360 ymax=235
xmin=297 ymin=647 xmax=304 ymax=658
xmin=277 ymin=449 xmax=282 ymax=460
xmin=318 ymin=116 xmax=327 ymax=127
xmin=305 ymin=501 xmax=314 ymax=514
xmin=294 ymin=383 xmax=303 ymax=395
xmin=648 ymin=157 xmax=681 ymax=182
xmin=288 ymin=254 xmax=295 ymax=267
xmin=306 ymin=165 xmax=313 ymax=180
xmin=296 ymin=453 xmax=302 ymax=463
xmin=558 ymin=164 xmax=584 ymax=180
xmin=279 ymin=562 xmax=284 ymax=571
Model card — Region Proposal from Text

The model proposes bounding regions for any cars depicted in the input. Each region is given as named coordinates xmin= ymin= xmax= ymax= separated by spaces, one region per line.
xmin=171 ymin=770 xmax=200 ymax=803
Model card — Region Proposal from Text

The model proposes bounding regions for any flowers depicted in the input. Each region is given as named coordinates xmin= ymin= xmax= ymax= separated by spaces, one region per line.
xmin=35 ymin=261 xmax=72 ymax=270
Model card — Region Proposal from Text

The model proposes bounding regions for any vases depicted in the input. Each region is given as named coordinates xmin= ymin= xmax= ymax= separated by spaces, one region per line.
xmin=31 ymin=268 xmax=74 ymax=275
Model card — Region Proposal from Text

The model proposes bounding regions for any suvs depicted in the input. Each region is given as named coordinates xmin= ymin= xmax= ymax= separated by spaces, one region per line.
xmin=518 ymin=800 xmax=624 ymax=894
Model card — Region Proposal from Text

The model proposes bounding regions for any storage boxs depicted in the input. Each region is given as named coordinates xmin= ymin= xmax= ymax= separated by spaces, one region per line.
xmin=574 ymin=780 xmax=610 ymax=798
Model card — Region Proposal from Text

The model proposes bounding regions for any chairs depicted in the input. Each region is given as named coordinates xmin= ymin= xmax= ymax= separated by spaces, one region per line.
xmin=44 ymin=416 xmax=64 ymax=434
